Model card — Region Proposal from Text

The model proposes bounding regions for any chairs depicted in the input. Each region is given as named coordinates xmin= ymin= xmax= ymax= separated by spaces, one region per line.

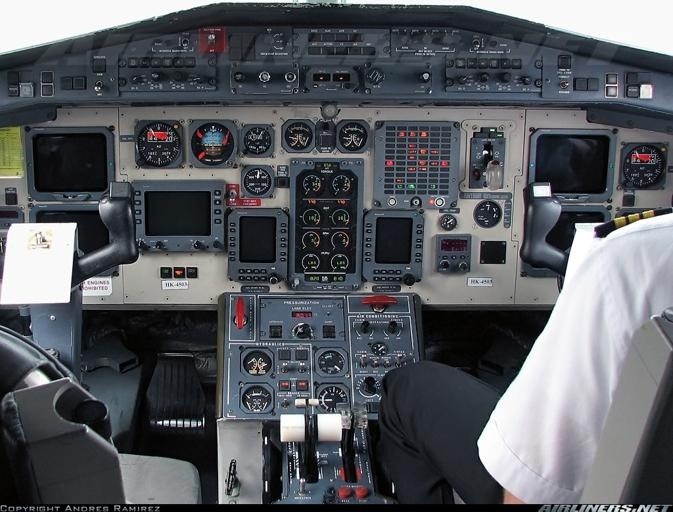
xmin=0 ymin=317 xmax=205 ymax=505
xmin=579 ymin=307 xmax=672 ymax=505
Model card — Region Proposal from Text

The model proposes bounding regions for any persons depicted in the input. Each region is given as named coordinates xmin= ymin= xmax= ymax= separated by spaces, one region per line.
xmin=377 ymin=206 xmax=673 ymax=504
